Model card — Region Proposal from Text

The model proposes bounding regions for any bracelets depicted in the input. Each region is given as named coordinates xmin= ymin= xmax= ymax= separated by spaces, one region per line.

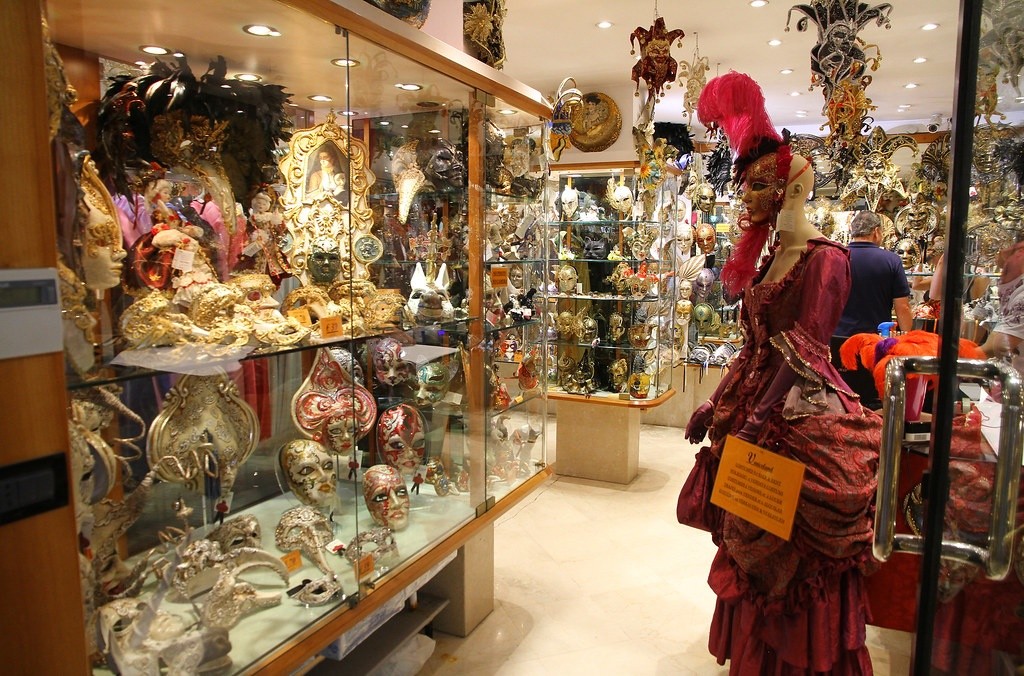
xmin=707 ymin=399 xmax=715 ymax=413
xmin=740 ymin=429 xmax=757 ymax=436
xmin=736 ymin=435 xmax=753 ymax=442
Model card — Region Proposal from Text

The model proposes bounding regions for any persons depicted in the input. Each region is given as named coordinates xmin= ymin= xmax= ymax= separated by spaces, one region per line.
xmin=677 ymin=153 xmax=882 ymax=676
xmin=830 ymin=210 xmax=913 ymax=412
xmin=912 ymin=240 xmax=1024 ymax=405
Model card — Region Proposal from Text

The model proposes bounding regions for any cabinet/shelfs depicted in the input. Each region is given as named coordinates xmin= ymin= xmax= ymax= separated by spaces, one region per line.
xmin=546 ymin=168 xmax=681 ymax=485
xmin=30 ymin=0 xmax=556 ymax=676
